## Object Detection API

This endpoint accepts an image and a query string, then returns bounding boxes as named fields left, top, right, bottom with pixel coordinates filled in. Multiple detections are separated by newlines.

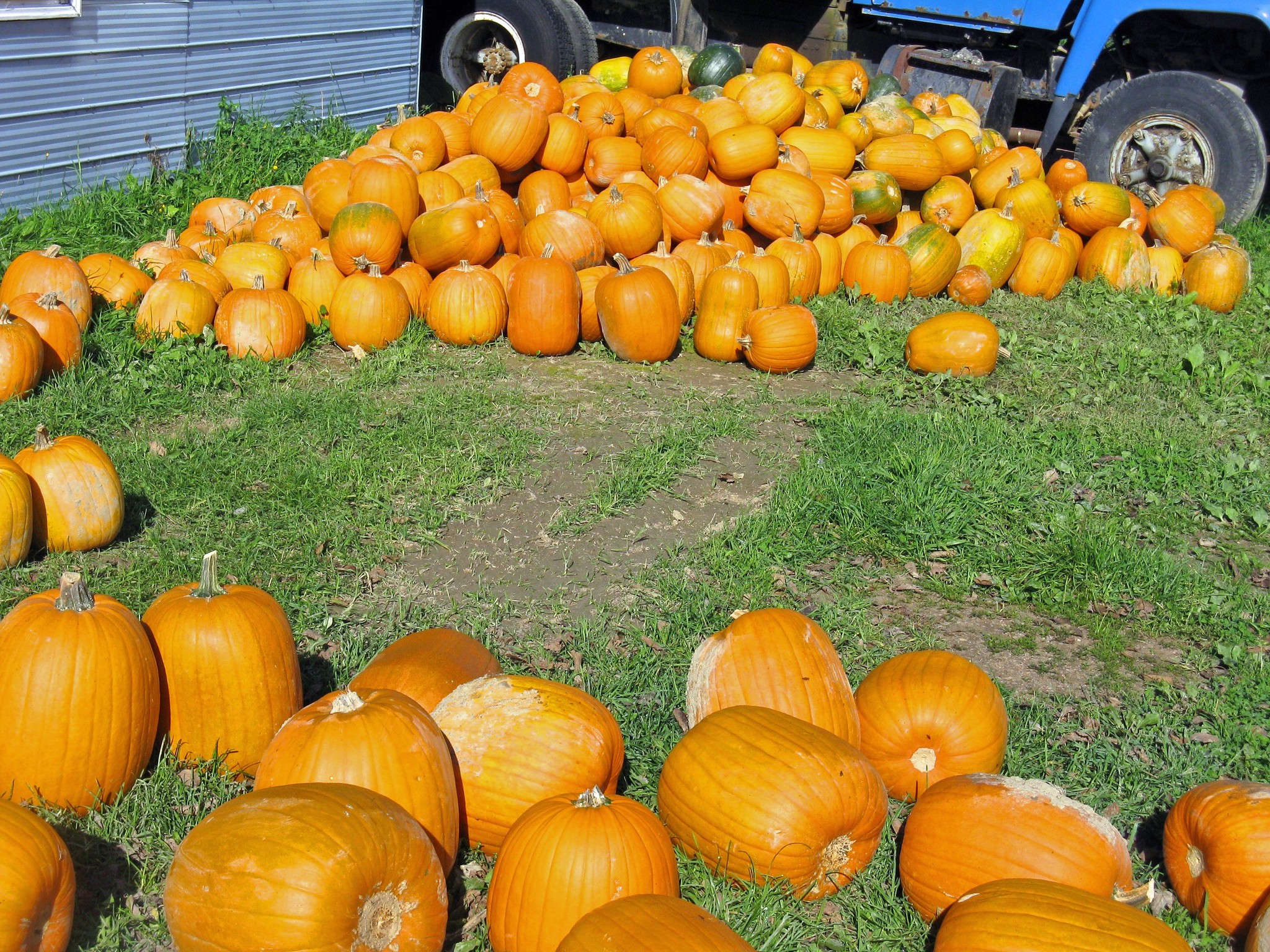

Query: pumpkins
left=428, top=675, right=627, bottom=857
left=656, top=708, right=889, bottom=901
left=140, top=549, right=303, bottom=790
left=12, top=424, right=127, bottom=558
left=161, top=781, right=448, bottom=951
left=933, top=879, right=1193, bottom=952
left=555, top=893, right=753, bottom=951
left=2, top=42, right=1256, bottom=412
left=2, top=803, right=77, bottom=952
left=347, top=627, right=503, bottom=716
left=685, top=608, right=863, bottom=751
left=0, top=453, right=34, bottom=572
left=853, top=648, right=1012, bottom=804
left=898, top=771, right=1157, bottom=927
left=254, top=688, right=463, bottom=877
left=2, top=574, right=164, bottom=823
left=486, top=784, right=681, bottom=951
left=1162, top=774, right=1269, bottom=951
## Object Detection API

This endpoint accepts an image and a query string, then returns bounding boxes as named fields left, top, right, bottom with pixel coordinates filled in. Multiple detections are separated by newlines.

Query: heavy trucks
left=422, top=1, right=1269, bottom=232
